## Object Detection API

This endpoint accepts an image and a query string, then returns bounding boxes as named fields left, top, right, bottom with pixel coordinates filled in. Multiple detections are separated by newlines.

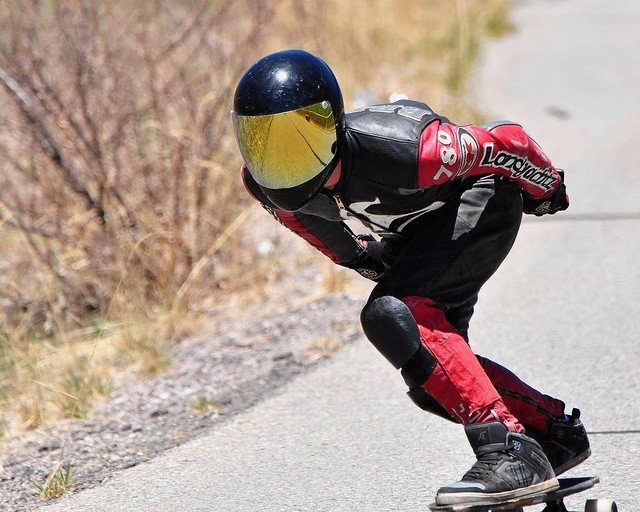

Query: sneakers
left=435, top=421, right=561, bottom=505
left=524, top=408, right=591, bottom=476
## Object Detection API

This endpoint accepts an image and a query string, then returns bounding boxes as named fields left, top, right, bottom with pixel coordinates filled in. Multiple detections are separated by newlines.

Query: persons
left=231, top=48, right=592, bottom=506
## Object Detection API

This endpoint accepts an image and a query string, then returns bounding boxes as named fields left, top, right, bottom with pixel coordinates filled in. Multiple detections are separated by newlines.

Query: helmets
left=231, top=48, right=347, bottom=213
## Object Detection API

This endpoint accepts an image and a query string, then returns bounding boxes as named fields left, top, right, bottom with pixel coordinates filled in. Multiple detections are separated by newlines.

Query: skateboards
left=428, top=476, right=618, bottom=512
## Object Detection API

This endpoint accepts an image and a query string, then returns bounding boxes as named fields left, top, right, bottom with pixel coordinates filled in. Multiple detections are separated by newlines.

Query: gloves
left=336, top=233, right=391, bottom=282
left=521, top=168, right=570, bottom=217
